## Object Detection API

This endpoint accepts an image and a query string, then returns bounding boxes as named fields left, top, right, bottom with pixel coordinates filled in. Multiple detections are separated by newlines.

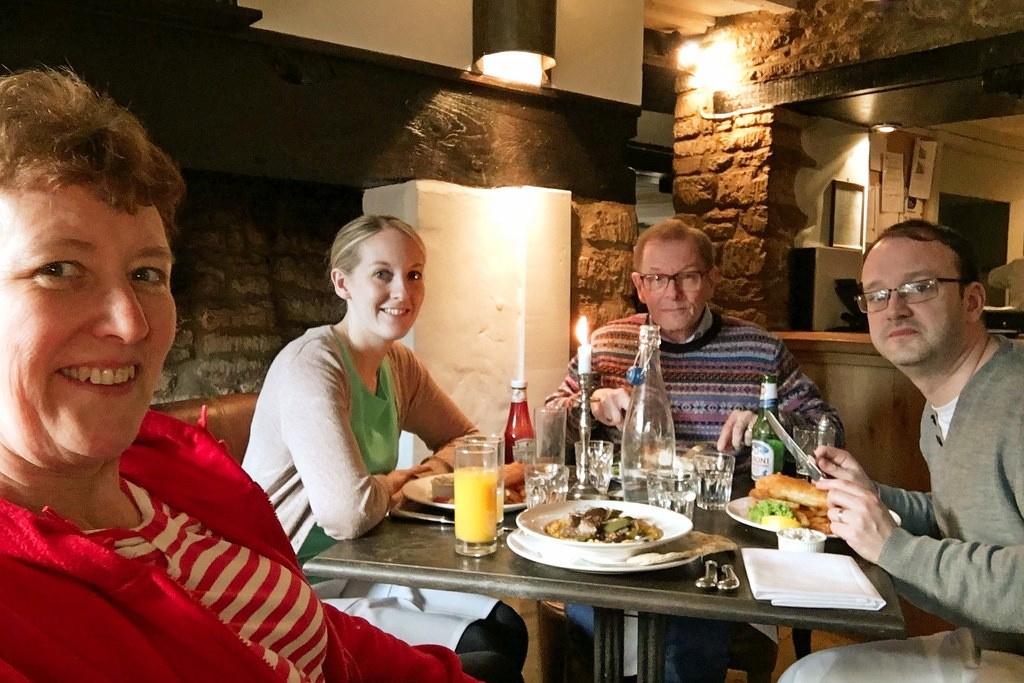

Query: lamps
left=472, top=0, right=557, bottom=87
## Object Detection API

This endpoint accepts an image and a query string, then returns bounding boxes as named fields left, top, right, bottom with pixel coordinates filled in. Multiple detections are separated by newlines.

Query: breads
left=749, top=474, right=828, bottom=507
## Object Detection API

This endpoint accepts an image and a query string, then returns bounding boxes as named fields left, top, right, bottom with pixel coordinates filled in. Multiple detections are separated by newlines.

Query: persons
left=0, top=70, right=515, bottom=683
left=778, top=219, right=1024, bottom=681
left=542, top=218, right=845, bottom=683
left=240, top=214, right=527, bottom=670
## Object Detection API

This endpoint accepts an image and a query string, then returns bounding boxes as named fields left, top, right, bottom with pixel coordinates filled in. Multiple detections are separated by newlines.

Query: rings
left=838, top=508, right=844, bottom=523
left=604, top=417, right=612, bottom=422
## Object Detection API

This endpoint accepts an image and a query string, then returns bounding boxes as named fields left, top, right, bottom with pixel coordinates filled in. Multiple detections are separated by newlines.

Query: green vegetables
left=747, top=501, right=793, bottom=523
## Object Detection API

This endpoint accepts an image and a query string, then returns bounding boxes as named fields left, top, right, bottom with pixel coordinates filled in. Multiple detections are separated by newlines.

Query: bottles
left=621, top=325, right=676, bottom=505
left=751, top=374, right=783, bottom=490
left=504, top=381, right=537, bottom=465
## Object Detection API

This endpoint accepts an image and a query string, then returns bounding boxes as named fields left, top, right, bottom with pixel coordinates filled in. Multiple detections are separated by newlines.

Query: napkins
left=742, top=547, right=886, bottom=610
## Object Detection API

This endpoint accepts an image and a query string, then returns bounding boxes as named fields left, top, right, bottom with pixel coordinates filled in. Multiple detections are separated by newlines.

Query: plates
left=726, top=496, right=901, bottom=537
left=402, top=472, right=527, bottom=513
left=609, top=456, right=697, bottom=483
left=506, top=527, right=700, bottom=574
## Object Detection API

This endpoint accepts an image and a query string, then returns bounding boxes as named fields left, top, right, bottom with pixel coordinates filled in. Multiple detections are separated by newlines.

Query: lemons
left=762, top=515, right=801, bottom=528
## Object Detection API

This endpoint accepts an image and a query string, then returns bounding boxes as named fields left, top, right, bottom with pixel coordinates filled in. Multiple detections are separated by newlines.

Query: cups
left=647, top=470, right=697, bottom=522
left=693, top=452, right=735, bottom=511
left=464, top=435, right=505, bottom=538
left=454, top=443, right=497, bottom=557
left=534, top=407, right=566, bottom=466
left=574, top=440, right=614, bottom=494
left=524, top=463, right=569, bottom=509
left=792, top=425, right=835, bottom=476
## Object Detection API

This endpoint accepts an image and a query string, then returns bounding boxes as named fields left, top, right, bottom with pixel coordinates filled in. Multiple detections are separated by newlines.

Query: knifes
left=388, top=508, right=516, bottom=535
left=764, top=410, right=827, bottom=483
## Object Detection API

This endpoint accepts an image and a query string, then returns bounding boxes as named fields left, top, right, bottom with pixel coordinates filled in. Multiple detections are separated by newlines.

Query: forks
left=795, top=430, right=820, bottom=464
left=683, top=442, right=717, bottom=458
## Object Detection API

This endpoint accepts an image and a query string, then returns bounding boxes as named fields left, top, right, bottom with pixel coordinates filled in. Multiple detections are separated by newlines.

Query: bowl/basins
left=776, top=527, right=826, bottom=553
left=430, top=476, right=454, bottom=502
left=516, top=500, right=693, bottom=562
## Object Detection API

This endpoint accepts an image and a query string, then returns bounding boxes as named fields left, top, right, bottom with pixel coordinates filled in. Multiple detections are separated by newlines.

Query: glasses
left=640, top=265, right=711, bottom=295
left=854, top=277, right=970, bottom=313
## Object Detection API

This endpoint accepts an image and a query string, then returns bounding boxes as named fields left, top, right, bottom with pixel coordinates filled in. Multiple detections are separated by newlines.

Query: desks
left=302, top=463, right=906, bottom=683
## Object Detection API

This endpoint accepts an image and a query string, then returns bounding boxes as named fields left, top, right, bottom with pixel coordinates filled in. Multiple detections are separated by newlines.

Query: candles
left=518, top=313, right=525, bottom=380
left=575, top=316, right=592, bottom=374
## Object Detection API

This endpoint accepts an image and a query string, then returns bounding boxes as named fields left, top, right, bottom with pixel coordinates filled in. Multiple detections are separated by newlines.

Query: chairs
left=148, top=393, right=258, bottom=467
